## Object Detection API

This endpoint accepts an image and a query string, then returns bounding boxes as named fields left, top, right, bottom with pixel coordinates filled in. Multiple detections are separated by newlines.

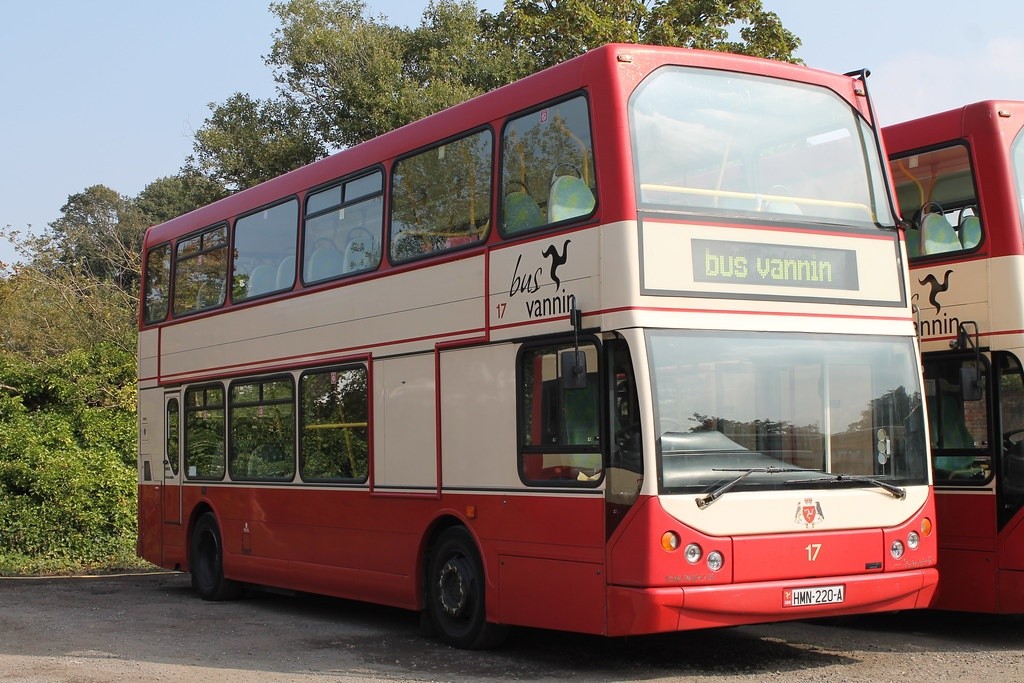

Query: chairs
left=276, top=245, right=299, bottom=290
left=902, top=218, right=919, bottom=259
left=219, top=277, right=227, bottom=307
left=546, top=163, right=597, bottom=227
left=307, top=247, right=334, bottom=285
left=194, top=278, right=218, bottom=310
left=957, top=202, right=981, bottom=252
left=342, top=227, right=378, bottom=274
left=761, top=185, right=800, bottom=214
left=244, top=260, right=278, bottom=298
left=919, top=198, right=963, bottom=258
left=504, top=179, right=544, bottom=241
left=928, top=396, right=985, bottom=481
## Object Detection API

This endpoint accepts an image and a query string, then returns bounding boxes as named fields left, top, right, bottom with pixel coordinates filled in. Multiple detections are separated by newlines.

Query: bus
left=526, top=94, right=1024, bottom=627
left=132, top=36, right=945, bottom=646
